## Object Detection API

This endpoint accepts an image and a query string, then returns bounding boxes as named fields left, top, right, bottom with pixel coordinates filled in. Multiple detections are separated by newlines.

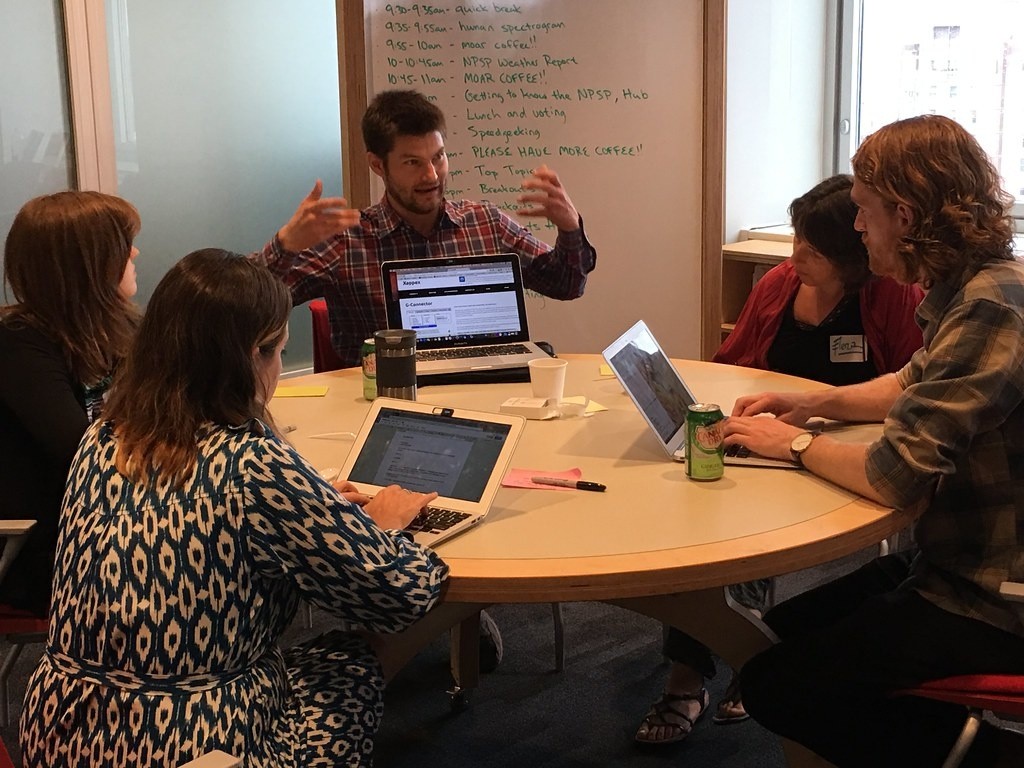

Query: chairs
left=300, top=299, right=565, bottom=673
left=0, top=517, right=50, bottom=768
left=893, top=581, right=1024, bottom=768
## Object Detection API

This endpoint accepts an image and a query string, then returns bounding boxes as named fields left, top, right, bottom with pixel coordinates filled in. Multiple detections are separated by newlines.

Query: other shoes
left=477, top=608, right=503, bottom=674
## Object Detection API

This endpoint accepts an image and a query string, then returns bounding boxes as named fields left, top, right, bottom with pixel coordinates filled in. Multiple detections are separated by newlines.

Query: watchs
left=790, top=429, right=822, bottom=470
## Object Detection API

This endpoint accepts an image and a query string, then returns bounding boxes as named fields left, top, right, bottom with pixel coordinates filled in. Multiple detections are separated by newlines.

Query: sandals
left=635, top=684, right=710, bottom=743
left=713, top=678, right=752, bottom=724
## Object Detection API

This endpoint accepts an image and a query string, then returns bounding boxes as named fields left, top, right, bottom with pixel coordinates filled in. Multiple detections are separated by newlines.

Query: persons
left=247, top=91, right=596, bottom=370
left=20, top=247, right=449, bottom=768
left=0, top=192, right=148, bottom=768
left=636, top=174, right=925, bottom=744
left=722, top=114, right=1024, bottom=768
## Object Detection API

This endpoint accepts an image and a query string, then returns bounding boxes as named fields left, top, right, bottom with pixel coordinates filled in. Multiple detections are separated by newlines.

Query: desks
left=265, top=354, right=934, bottom=768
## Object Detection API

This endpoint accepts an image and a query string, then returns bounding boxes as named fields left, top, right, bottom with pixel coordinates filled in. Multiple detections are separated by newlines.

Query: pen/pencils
left=531, top=475, right=608, bottom=491
left=282, top=424, right=297, bottom=434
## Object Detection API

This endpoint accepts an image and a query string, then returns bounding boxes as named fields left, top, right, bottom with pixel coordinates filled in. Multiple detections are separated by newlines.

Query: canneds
left=685, top=403, right=725, bottom=481
left=362, top=338, right=378, bottom=401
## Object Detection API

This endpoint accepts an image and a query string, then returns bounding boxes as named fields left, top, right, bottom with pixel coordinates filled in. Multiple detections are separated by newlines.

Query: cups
left=374, top=329, right=417, bottom=401
left=527, top=358, right=568, bottom=398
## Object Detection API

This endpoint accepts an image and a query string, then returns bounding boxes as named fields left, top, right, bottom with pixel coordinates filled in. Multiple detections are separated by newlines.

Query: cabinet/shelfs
left=721, top=239, right=793, bottom=333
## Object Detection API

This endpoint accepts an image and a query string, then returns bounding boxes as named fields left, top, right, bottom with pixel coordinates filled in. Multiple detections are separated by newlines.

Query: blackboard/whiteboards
left=336, top=0, right=728, bottom=362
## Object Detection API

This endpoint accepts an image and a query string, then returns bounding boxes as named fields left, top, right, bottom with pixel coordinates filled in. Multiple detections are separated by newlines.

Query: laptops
left=335, top=397, right=526, bottom=550
left=380, top=252, right=550, bottom=376
left=602, top=320, right=825, bottom=469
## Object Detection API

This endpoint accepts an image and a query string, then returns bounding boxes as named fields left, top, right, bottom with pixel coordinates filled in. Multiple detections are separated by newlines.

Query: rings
left=402, top=488, right=412, bottom=495
